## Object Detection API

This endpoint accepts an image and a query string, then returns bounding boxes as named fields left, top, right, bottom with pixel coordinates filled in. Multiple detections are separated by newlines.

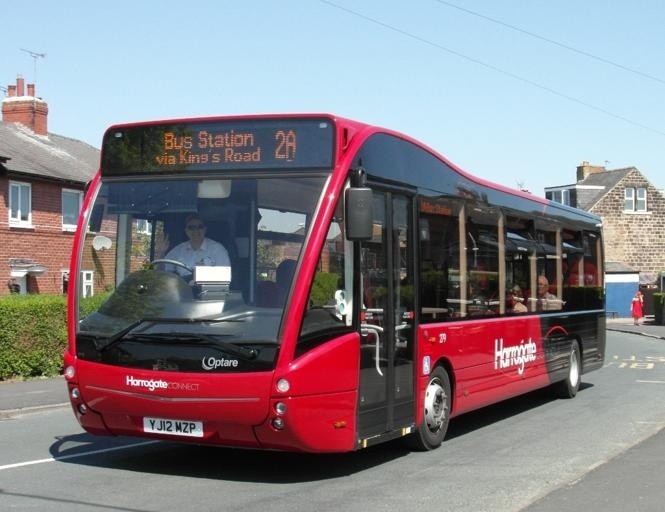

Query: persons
left=506, top=283, right=530, bottom=314
left=159, top=210, right=231, bottom=288
left=526, top=276, right=561, bottom=312
left=7, top=278, right=21, bottom=294
left=468, top=289, right=497, bottom=318
left=630, top=291, right=644, bottom=326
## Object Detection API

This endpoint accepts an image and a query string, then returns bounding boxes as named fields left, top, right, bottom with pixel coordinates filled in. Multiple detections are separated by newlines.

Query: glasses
left=187, top=224, right=206, bottom=230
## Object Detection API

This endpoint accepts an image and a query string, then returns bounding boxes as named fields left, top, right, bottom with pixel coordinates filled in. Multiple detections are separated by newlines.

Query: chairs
left=521, top=256, right=598, bottom=311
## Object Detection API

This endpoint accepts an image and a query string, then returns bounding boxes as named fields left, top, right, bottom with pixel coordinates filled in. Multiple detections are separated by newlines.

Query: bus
left=61, top=110, right=610, bottom=460
